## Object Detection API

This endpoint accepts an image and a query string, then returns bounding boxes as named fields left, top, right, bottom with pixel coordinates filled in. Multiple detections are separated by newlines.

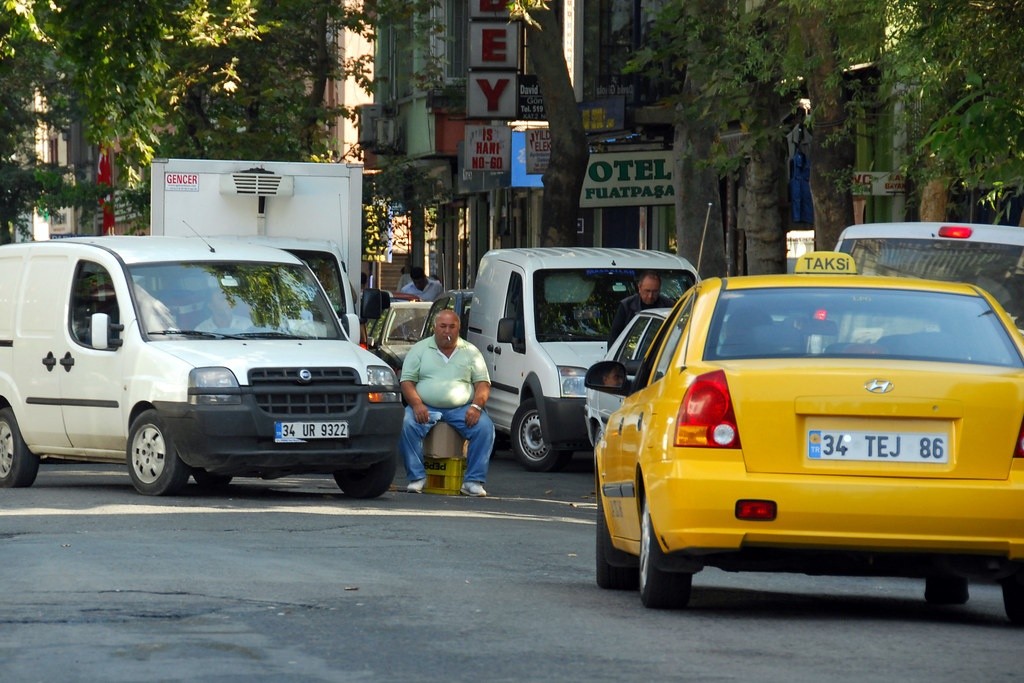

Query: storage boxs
left=422, top=421, right=469, bottom=495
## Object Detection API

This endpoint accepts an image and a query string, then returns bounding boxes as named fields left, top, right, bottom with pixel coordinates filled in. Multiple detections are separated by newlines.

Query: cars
left=581, top=250, right=1024, bottom=627
left=365, top=302, right=432, bottom=405
left=418, top=288, right=474, bottom=343
left=582, top=307, right=686, bottom=452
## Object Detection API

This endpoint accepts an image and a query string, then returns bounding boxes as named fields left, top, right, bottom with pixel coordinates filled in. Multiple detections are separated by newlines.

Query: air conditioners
left=356, top=102, right=382, bottom=145
left=373, top=117, right=395, bottom=147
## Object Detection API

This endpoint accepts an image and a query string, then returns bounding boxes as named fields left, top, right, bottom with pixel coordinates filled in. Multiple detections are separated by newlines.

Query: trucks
left=150, top=153, right=365, bottom=351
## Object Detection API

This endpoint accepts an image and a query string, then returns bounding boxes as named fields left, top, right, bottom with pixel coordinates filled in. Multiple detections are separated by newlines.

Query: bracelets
left=471, top=404, right=482, bottom=412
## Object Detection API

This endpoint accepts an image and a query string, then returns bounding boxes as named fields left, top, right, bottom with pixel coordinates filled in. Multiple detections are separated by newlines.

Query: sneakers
left=460, top=481, right=486, bottom=496
left=407, top=478, right=425, bottom=493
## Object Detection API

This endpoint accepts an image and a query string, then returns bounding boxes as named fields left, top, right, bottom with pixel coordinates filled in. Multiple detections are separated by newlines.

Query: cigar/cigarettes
left=448, top=335, right=451, bottom=341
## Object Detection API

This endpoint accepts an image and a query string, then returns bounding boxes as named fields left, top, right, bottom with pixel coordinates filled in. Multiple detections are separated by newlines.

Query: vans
left=0, top=236, right=407, bottom=501
left=831, top=222, right=1023, bottom=351
left=466, top=247, right=703, bottom=475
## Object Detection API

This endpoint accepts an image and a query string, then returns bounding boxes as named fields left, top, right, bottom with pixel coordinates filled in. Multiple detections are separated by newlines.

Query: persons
left=194, top=288, right=254, bottom=334
left=398, top=309, right=495, bottom=495
left=397, top=267, right=444, bottom=302
left=602, top=365, right=624, bottom=386
left=608, top=273, right=673, bottom=352
left=360, top=272, right=366, bottom=344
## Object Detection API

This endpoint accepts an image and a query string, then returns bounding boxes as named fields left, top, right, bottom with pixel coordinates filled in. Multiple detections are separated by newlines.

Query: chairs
left=721, top=307, right=782, bottom=357
left=774, top=316, right=838, bottom=354
left=825, top=342, right=890, bottom=357
left=86, top=282, right=117, bottom=319
